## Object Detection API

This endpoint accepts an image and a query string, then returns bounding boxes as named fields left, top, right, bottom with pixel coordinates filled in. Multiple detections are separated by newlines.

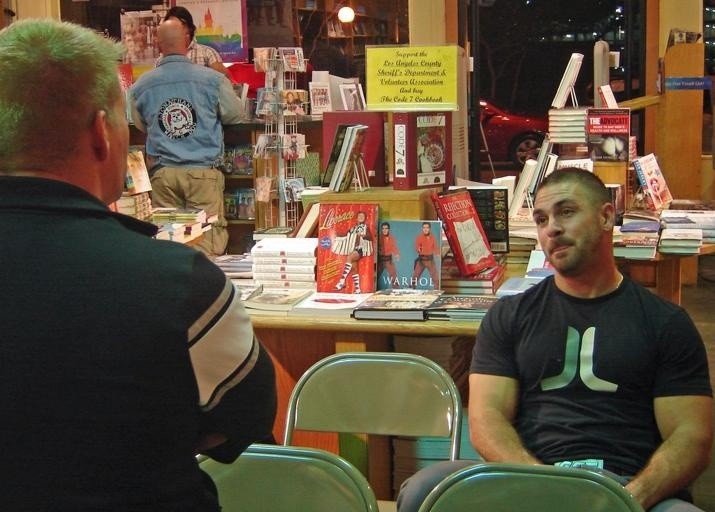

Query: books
left=338, top=83, right=368, bottom=110
left=303, top=1, right=387, bottom=58
left=253, top=45, right=309, bottom=206
left=549, top=51, right=585, bottom=109
left=336, top=331, right=485, bottom=511
left=307, top=81, right=333, bottom=115
left=196, top=101, right=715, bottom=322
left=104, top=150, right=219, bottom=243
left=597, top=84, right=621, bottom=109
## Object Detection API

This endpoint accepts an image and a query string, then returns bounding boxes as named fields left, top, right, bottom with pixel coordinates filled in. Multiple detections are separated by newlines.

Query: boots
left=334, top=264, right=351, bottom=291
left=353, top=274, right=360, bottom=293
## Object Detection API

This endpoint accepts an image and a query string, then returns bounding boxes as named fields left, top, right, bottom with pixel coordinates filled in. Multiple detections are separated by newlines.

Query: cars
left=478, top=93, right=550, bottom=172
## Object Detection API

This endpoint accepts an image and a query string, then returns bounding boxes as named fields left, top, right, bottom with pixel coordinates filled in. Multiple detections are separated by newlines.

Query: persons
left=126, top=18, right=247, bottom=257
left=395, top=168, right=715, bottom=510
left=154, top=5, right=228, bottom=77
left=0, top=14, right=279, bottom=511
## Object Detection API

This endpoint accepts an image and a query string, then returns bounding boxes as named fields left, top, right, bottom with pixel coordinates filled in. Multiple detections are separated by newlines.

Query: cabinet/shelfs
left=220, top=121, right=266, bottom=229
left=294, top=1, right=401, bottom=77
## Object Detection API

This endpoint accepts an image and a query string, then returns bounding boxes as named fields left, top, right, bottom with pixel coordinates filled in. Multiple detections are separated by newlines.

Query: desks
left=248, top=259, right=532, bottom=498
